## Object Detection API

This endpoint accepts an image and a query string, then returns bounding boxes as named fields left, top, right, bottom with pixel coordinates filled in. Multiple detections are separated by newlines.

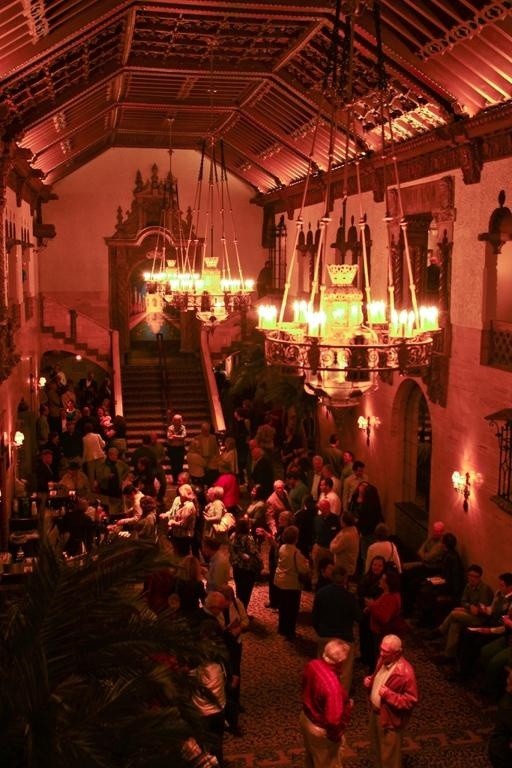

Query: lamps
left=32, top=221, right=58, bottom=255
left=450, top=467, right=476, bottom=514
left=357, top=412, right=379, bottom=448
left=33, top=375, right=48, bottom=391
left=167, top=34, right=262, bottom=336
left=10, top=430, right=29, bottom=453
left=140, top=112, right=201, bottom=302
left=254, top=2, right=446, bottom=422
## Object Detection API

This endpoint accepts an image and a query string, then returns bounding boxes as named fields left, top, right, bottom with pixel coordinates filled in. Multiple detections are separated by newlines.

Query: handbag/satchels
left=300, top=573, right=313, bottom=583
left=213, top=511, right=238, bottom=534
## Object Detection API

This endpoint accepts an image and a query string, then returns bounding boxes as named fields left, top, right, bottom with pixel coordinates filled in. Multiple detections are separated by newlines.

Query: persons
left=487, top=664, right=511, bottom=768
left=297, top=639, right=353, bottom=768
left=363, top=635, right=418, bottom=768
left=0, top=361, right=511, bottom=737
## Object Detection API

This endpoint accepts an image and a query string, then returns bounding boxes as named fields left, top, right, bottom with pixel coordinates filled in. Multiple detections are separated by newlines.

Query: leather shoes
left=432, top=653, right=454, bottom=665
left=426, top=626, right=443, bottom=639
left=231, top=726, right=244, bottom=738
